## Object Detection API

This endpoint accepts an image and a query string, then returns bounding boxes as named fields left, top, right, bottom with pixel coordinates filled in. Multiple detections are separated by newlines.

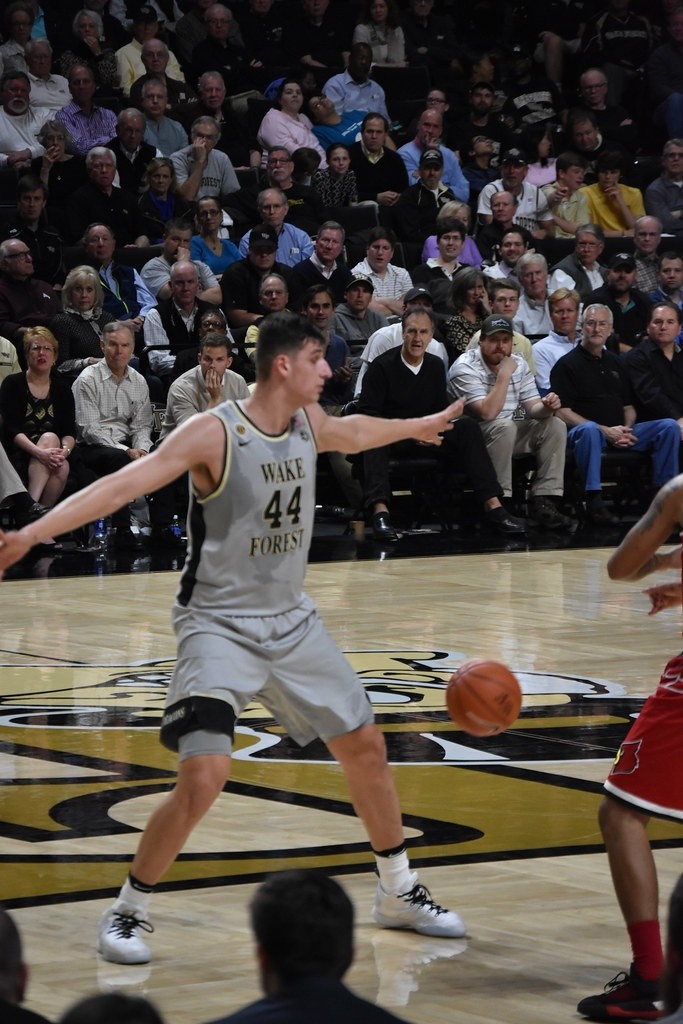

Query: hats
left=132, top=5, right=157, bottom=24
left=345, top=275, right=374, bottom=293
left=420, top=149, right=443, bottom=166
left=481, top=314, right=513, bottom=338
left=501, top=148, right=526, bottom=164
left=404, top=288, right=434, bottom=305
left=608, top=252, right=636, bottom=270
left=250, top=224, right=278, bottom=247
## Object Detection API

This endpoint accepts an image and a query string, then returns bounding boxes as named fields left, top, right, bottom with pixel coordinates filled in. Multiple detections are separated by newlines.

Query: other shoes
left=149, top=523, right=172, bottom=553
left=11, top=502, right=52, bottom=531
left=527, top=503, right=578, bottom=531
left=113, top=529, right=142, bottom=551
left=585, top=503, right=619, bottom=528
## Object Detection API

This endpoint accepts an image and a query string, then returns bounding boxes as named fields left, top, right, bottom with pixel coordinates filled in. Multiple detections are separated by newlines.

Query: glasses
left=426, top=98, right=446, bottom=105
left=313, top=95, right=327, bottom=109
left=611, top=263, right=634, bottom=273
left=201, top=321, right=225, bottom=329
left=268, top=157, right=292, bottom=165
left=199, top=209, right=220, bottom=218
left=3, top=250, right=31, bottom=260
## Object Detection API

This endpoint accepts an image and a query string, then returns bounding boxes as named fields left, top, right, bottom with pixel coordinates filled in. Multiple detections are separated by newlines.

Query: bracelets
left=62, top=446, right=71, bottom=455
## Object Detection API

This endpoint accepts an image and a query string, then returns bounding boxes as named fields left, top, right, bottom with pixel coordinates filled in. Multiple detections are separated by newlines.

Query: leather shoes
left=375, top=513, right=396, bottom=538
left=482, top=515, right=525, bottom=534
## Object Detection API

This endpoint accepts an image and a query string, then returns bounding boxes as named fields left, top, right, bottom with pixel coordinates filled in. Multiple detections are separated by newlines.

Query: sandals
left=37, top=540, right=64, bottom=550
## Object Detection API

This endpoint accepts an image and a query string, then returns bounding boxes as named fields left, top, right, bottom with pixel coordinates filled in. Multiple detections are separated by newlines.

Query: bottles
left=95, top=516, right=108, bottom=561
left=169, top=514, right=182, bottom=541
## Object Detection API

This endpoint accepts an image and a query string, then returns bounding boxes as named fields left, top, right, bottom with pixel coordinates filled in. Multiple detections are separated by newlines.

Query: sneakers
left=96, top=902, right=154, bottom=964
left=576, top=963, right=679, bottom=1024
left=98, top=958, right=151, bottom=991
left=371, top=929, right=467, bottom=985
left=371, top=868, right=467, bottom=938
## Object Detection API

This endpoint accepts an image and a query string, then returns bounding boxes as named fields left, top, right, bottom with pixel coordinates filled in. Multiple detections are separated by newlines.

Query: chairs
left=338, top=451, right=650, bottom=530
left=383, top=66, right=432, bottom=126
left=0, top=143, right=411, bottom=274
left=530, top=234, right=683, bottom=270
left=624, top=155, right=661, bottom=196
left=621, top=65, right=670, bottom=155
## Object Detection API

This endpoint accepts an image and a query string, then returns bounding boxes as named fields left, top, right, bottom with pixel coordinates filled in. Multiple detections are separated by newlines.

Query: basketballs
left=445, top=659, right=522, bottom=736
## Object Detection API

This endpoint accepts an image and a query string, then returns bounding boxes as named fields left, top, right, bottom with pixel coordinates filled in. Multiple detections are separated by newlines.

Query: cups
left=350, top=521, right=365, bottom=536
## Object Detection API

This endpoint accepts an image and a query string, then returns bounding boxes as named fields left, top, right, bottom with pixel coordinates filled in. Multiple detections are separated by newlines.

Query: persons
left=0, top=0, right=683, bottom=558
left=0, top=311, right=466, bottom=965
left=576, top=474, right=683, bottom=1024
left=0, top=869, right=411, bottom=1024
